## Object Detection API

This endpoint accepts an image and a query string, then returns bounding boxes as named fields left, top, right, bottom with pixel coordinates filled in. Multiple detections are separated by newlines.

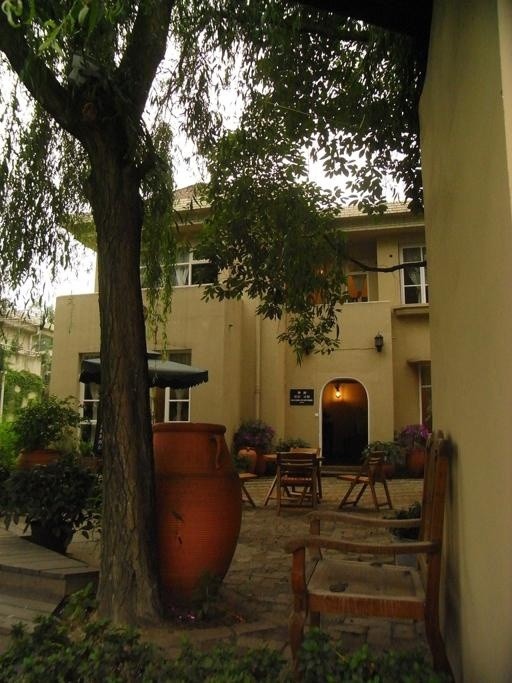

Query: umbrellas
left=78, top=351, right=208, bottom=390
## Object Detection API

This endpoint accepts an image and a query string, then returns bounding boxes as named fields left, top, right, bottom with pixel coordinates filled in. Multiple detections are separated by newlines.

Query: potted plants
left=381, top=499, right=422, bottom=566
left=363, top=439, right=401, bottom=478
left=1, top=452, right=105, bottom=554
left=11, top=392, right=91, bottom=470
left=77, top=439, right=98, bottom=470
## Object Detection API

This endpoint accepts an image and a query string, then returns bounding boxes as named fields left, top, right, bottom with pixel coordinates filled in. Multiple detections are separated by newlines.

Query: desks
left=261, top=453, right=328, bottom=506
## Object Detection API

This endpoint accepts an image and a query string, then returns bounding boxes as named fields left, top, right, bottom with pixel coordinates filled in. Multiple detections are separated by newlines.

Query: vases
left=405, top=448, right=428, bottom=478
left=236, top=447, right=259, bottom=473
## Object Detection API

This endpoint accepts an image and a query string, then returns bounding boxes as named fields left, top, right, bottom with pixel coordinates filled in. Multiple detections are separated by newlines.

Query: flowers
left=394, top=423, right=430, bottom=447
left=233, top=418, right=276, bottom=447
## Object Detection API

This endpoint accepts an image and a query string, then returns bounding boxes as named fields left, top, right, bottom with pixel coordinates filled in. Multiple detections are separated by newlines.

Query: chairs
left=238, top=471, right=259, bottom=507
left=289, top=445, right=320, bottom=500
left=286, top=428, right=453, bottom=681
left=275, top=451, right=319, bottom=516
left=335, top=449, right=394, bottom=511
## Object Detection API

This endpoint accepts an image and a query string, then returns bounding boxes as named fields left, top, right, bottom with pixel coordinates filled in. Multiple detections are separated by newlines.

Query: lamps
left=333, top=381, right=343, bottom=397
left=375, top=329, right=384, bottom=352
